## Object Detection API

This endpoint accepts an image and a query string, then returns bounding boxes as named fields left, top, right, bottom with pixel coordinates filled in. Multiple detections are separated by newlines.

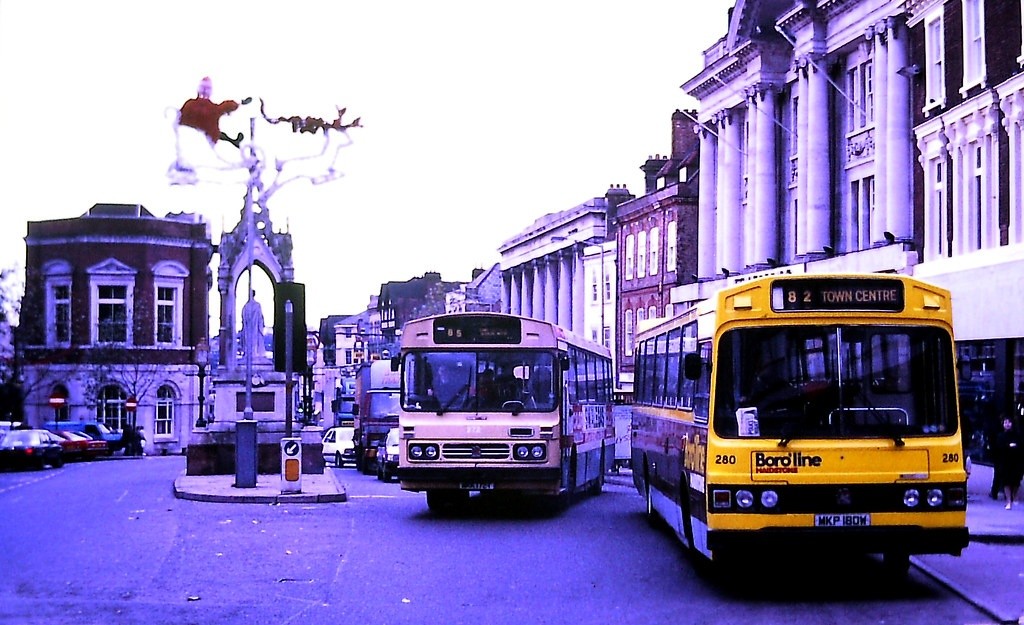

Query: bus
left=630, top=274, right=971, bottom=579
left=389, top=311, right=616, bottom=514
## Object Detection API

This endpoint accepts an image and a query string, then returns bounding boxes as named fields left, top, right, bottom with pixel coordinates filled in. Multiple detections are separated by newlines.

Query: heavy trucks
left=332, top=381, right=358, bottom=426
left=353, top=361, right=403, bottom=475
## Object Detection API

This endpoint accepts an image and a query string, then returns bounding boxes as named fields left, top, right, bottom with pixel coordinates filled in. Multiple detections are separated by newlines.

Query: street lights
left=186, top=337, right=217, bottom=476
left=549, top=236, right=604, bottom=345
left=298, top=352, right=323, bottom=475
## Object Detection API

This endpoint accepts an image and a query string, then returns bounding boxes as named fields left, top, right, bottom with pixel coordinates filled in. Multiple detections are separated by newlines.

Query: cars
left=51, top=430, right=109, bottom=461
left=1, top=429, right=64, bottom=472
left=322, top=427, right=358, bottom=467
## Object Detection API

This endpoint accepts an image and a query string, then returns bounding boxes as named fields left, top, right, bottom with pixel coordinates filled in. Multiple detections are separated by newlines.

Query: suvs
left=376, top=428, right=403, bottom=483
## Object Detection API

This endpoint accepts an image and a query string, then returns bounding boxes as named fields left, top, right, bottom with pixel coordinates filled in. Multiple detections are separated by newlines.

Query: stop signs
left=125, top=397, right=137, bottom=412
left=48, top=390, right=65, bottom=408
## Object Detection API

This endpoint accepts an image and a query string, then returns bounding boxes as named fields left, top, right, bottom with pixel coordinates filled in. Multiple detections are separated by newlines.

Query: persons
left=240, top=290, right=268, bottom=359
left=428, top=358, right=552, bottom=407
left=986, top=415, right=1024, bottom=510
left=121, top=424, right=148, bottom=456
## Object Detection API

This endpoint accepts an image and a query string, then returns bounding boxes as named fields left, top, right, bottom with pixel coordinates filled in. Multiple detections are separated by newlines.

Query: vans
left=46, top=421, right=124, bottom=457
